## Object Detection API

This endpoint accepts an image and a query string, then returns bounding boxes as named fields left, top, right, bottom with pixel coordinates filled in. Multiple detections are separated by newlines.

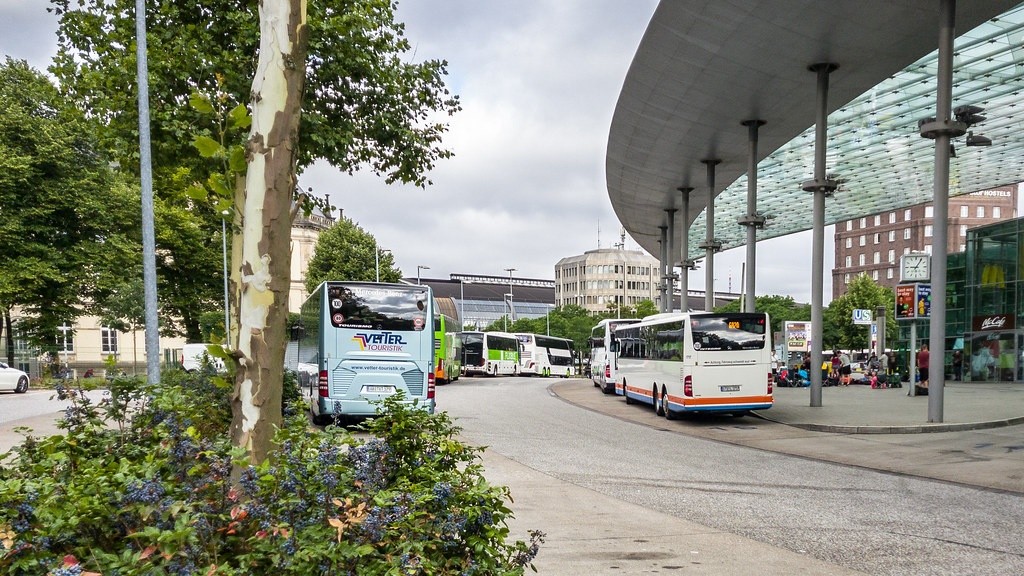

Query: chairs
left=788, top=368, right=803, bottom=387
left=822, top=369, right=829, bottom=387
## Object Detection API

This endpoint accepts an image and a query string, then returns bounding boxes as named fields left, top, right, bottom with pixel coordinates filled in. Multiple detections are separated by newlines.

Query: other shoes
left=846, top=383, right=849, bottom=387
left=841, top=383, right=844, bottom=386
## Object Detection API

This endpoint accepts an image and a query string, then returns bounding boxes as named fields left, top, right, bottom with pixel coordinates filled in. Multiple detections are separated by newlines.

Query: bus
left=514, top=333, right=576, bottom=378
left=463, top=332, right=525, bottom=377
left=435, top=315, right=463, bottom=384
left=590, top=308, right=774, bottom=421
left=298, top=280, right=436, bottom=427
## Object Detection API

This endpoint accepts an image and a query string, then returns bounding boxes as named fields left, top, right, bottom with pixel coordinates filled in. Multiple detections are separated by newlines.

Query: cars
left=0, top=362, right=30, bottom=394
left=584, top=357, right=591, bottom=378
left=849, top=362, right=868, bottom=383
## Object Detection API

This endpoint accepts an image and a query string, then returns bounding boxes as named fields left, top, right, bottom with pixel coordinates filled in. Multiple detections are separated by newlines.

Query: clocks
left=900, top=253, right=930, bottom=282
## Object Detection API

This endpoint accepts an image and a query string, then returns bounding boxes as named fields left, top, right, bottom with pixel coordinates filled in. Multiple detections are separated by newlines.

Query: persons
left=805, top=354, right=811, bottom=381
left=918, top=344, right=929, bottom=387
left=952, top=351, right=962, bottom=381
left=919, top=297, right=925, bottom=315
left=897, top=295, right=908, bottom=317
left=830, top=349, right=852, bottom=386
left=771, top=350, right=787, bottom=385
left=84, top=368, right=94, bottom=378
left=864, top=352, right=897, bottom=388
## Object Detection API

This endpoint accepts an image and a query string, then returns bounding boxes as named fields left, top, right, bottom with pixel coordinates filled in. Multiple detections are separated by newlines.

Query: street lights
left=418, top=265, right=431, bottom=285
left=376, top=246, right=392, bottom=282
left=458, top=277, right=471, bottom=330
left=504, top=292, right=514, bottom=333
left=221, top=210, right=230, bottom=349
left=504, top=268, right=518, bottom=325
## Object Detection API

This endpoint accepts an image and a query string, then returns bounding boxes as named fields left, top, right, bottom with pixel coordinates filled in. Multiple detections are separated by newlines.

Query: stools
left=876, top=380, right=887, bottom=389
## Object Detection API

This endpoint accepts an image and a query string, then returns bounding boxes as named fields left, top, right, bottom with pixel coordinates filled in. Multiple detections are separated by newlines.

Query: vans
left=182, top=342, right=231, bottom=375
